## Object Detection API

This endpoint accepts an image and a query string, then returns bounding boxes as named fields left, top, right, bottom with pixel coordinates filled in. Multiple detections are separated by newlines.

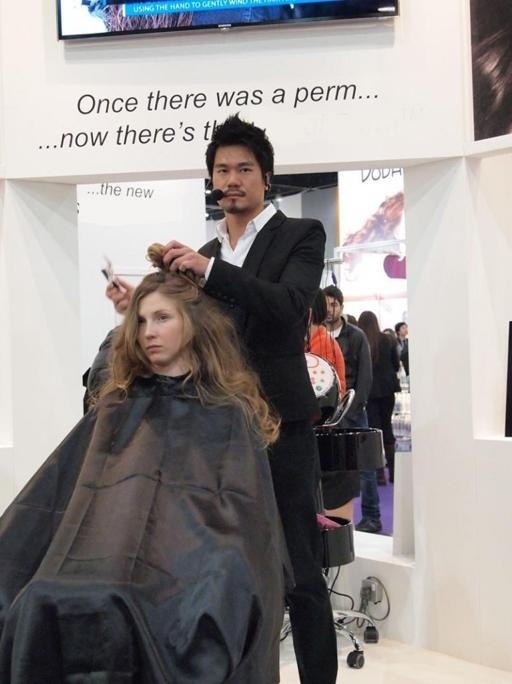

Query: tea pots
left=77, top=167, right=411, bottom=536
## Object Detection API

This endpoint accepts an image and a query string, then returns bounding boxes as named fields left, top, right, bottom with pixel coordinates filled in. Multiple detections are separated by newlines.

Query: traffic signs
left=360, top=580, right=384, bottom=605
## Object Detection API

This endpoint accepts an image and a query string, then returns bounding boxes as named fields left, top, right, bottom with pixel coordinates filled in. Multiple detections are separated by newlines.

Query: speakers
left=384, top=444, right=397, bottom=483
left=377, top=468, right=386, bottom=486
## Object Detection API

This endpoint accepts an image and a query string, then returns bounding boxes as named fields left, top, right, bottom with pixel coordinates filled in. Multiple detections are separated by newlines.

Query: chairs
left=355, top=521, right=381, bottom=532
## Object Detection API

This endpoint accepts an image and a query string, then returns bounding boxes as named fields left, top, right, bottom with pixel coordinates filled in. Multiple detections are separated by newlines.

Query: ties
left=55, top=0, right=400, bottom=41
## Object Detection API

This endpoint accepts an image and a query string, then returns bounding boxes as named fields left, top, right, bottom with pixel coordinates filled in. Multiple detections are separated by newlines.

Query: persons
left=305, top=286, right=409, bottom=532
left=0, top=243, right=296, bottom=684
left=106, top=113, right=338, bottom=684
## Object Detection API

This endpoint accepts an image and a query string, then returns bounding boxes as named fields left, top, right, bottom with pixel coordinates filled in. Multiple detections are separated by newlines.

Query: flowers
left=205, top=188, right=227, bottom=201
